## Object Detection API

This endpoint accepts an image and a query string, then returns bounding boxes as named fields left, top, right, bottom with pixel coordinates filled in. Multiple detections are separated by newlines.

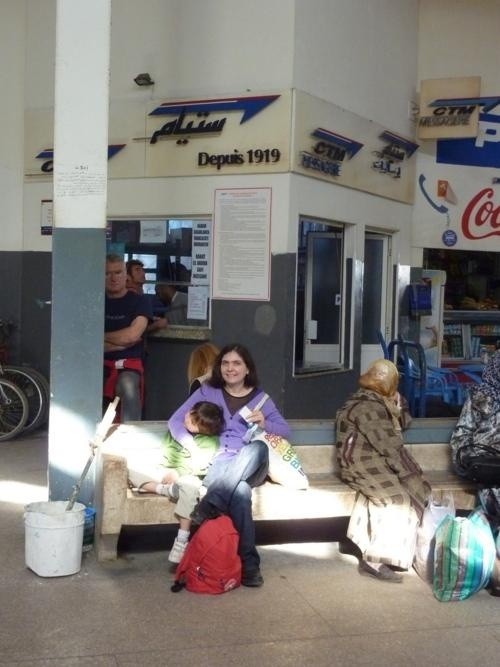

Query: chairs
left=378, top=330, right=463, bottom=409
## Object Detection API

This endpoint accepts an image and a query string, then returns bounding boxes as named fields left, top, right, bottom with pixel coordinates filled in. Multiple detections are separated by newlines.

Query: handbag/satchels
left=250, top=429, right=310, bottom=490
left=467, top=455, right=500, bottom=488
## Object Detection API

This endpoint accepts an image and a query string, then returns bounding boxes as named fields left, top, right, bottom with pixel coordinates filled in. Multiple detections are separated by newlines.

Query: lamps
left=134, top=72, right=152, bottom=86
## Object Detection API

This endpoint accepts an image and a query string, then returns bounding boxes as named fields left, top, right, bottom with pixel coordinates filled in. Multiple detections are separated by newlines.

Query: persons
left=126, top=402, right=224, bottom=562
left=124, top=259, right=147, bottom=296
left=102, top=253, right=154, bottom=423
left=187, top=343, right=222, bottom=398
left=153, top=277, right=189, bottom=324
left=166, top=342, right=291, bottom=587
left=332, top=360, right=432, bottom=582
left=449, top=348, right=500, bottom=592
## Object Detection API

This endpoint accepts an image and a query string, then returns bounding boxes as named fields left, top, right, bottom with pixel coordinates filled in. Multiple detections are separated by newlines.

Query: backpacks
left=175, top=511, right=242, bottom=596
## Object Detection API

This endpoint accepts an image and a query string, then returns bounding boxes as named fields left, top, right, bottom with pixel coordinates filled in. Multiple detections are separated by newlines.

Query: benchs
left=94, top=414, right=482, bottom=562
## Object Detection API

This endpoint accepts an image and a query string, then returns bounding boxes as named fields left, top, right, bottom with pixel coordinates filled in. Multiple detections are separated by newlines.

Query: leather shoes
left=359, top=559, right=404, bottom=583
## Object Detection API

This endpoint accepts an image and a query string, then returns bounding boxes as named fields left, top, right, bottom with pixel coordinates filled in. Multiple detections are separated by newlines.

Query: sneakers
left=167, top=536, right=189, bottom=564
left=166, top=483, right=180, bottom=502
left=241, top=569, right=263, bottom=587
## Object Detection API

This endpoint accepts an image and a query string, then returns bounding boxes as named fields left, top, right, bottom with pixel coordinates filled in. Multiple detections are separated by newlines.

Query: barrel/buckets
left=24, top=500, right=86, bottom=577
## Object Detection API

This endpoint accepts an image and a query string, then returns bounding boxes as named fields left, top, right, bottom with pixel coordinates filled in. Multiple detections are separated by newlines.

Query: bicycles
left=0, top=319, right=50, bottom=444
